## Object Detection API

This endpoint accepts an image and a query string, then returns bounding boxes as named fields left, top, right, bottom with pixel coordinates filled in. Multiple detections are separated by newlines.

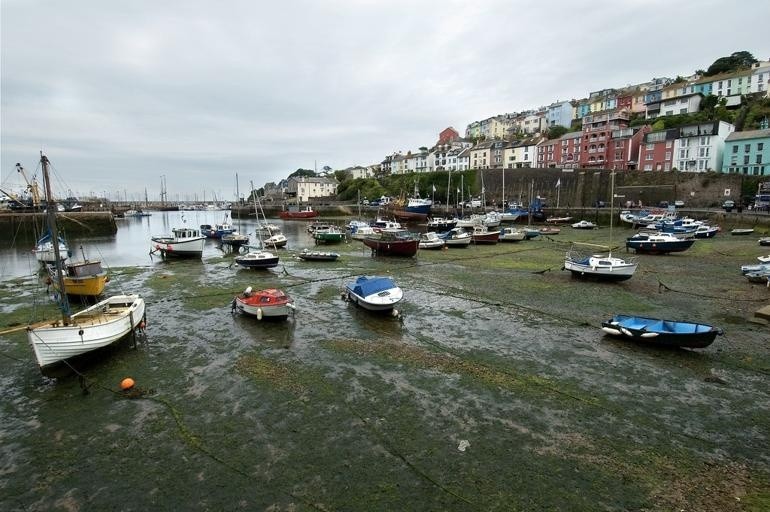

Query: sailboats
left=25, top=156, right=147, bottom=380
left=563, top=164, right=640, bottom=280
left=0, top=150, right=287, bottom=272
left=345, top=165, right=574, bottom=238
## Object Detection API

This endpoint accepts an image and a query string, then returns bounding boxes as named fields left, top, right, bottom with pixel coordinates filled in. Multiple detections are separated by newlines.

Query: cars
left=592, top=200, right=604, bottom=208
left=674, top=200, right=685, bottom=208
left=655, top=201, right=668, bottom=208
left=623, top=200, right=642, bottom=208
left=722, top=200, right=735, bottom=209
left=747, top=203, right=753, bottom=211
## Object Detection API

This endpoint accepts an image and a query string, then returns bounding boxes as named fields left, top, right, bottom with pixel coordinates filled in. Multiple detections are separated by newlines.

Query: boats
left=278, top=196, right=317, bottom=219
left=436, top=225, right=471, bottom=248
left=497, top=226, right=527, bottom=242
left=467, top=217, right=501, bottom=245
left=299, top=248, right=339, bottom=262
left=362, top=224, right=421, bottom=257
left=418, top=231, right=445, bottom=251
left=342, top=274, right=404, bottom=311
left=731, top=228, right=754, bottom=235
left=619, top=207, right=720, bottom=258
left=741, top=236, right=770, bottom=289
left=234, top=286, right=294, bottom=319
left=601, top=311, right=724, bottom=350
left=49, top=243, right=108, bottom=298
left=305, top=219, right=347, bottom=245
left=571, top=219, right=597, bottom=230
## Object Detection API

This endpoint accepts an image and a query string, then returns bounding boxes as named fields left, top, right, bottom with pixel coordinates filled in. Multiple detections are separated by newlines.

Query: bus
left=753, top=194, right=770, bottom=211
